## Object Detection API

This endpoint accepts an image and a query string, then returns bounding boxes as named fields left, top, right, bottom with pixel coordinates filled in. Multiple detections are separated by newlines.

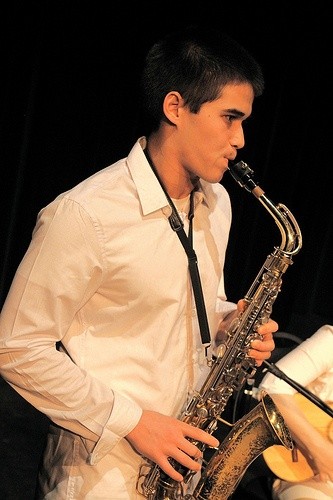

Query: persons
left=255, top=324, right=332, bottom=499
left=0, top=37, right=279, bottom=500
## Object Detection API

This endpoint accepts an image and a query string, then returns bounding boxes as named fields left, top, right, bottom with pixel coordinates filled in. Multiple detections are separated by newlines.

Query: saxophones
left=135, top=159, right=294, bottom=500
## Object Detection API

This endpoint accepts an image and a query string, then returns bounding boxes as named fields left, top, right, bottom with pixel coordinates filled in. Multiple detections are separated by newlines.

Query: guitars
left=246, top=393, right=333, bottom=483
